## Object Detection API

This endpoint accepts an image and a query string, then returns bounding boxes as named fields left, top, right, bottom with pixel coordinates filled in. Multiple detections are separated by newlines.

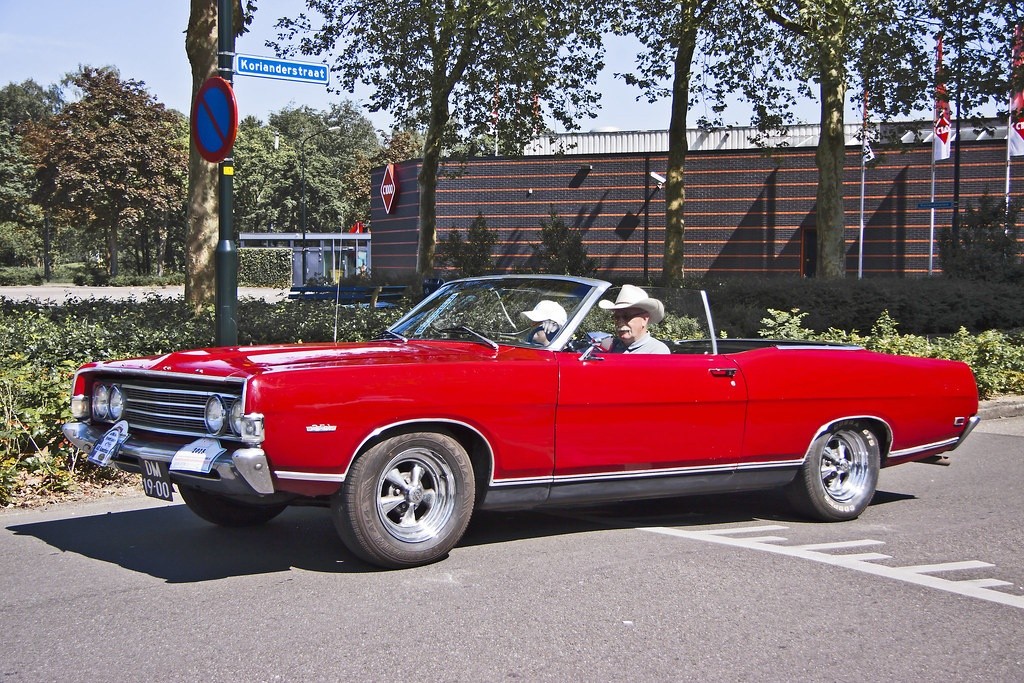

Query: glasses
left=529, top=322, right=542, bottom=327
left=610, top=311, right=648, bottom=323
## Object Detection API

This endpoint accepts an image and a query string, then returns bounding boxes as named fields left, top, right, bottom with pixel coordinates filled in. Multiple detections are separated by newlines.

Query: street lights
left=301, top=126, right=341, bottom=288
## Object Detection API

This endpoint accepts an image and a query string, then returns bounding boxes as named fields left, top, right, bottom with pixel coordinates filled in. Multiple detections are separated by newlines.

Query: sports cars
left=64, top=274, right=982, bottom=567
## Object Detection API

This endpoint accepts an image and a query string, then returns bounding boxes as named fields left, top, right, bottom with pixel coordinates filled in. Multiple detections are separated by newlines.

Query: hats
left=520, top=300, right=567, bottom=327
left=599, top=284, right=665, bottom=324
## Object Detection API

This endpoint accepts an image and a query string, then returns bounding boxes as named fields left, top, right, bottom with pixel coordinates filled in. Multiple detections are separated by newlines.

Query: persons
left=519, top=300, right=567, bottom=348
left=598, top=284, right=674, bottom=354
